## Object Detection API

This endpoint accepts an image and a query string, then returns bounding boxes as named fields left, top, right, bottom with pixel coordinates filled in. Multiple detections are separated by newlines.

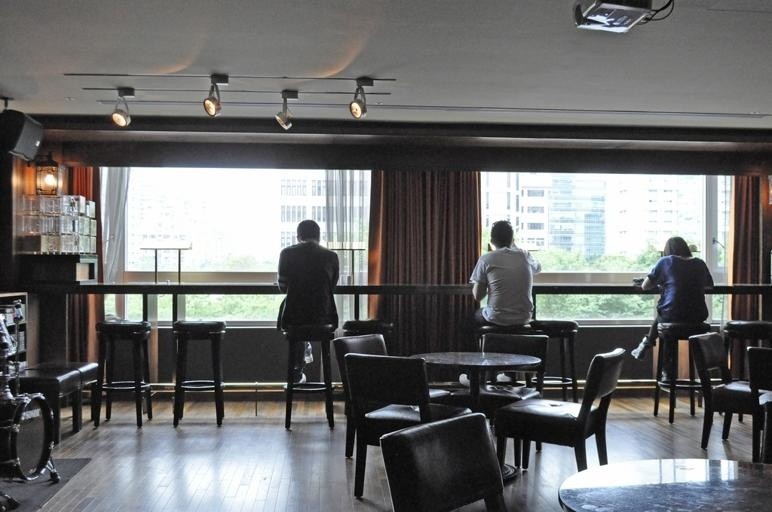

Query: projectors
left=571, top=0, right=654, bottom=36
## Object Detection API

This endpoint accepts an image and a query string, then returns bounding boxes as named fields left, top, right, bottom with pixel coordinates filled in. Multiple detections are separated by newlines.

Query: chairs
left=495, top=348, right=626, bottom=473
left=747, top=346, right=772, bottom=462
left=344, top=352, right=471, bottom=498
left=689, top=332, right=767, bottom=448
left=379, top=412, right=507, bottom=511
left=449, top=332, right=549, bottom=451
left=333, top=333, right=449, bottom=459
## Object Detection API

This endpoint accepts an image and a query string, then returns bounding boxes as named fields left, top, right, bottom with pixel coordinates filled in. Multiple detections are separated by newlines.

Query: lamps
left=113, top=87, right=137, bottom=126
left=349, top=77, right=373, bottom=118
left=275, top=90, right=300, bottom=130
left=25, top=153, right=64, bottom=195
left=203, top=72, right=230, bottom=116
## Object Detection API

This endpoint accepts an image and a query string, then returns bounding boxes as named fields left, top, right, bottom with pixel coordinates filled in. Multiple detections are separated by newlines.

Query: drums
left=0, top=314, right=16, bottom=361
left=0, top=391, right=60, bottom=484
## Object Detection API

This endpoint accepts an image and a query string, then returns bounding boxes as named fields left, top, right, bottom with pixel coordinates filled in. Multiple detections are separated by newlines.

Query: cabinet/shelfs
left=0, top=292, right=27, bottom=381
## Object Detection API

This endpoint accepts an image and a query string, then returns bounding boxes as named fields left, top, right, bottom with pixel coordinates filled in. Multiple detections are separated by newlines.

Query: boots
left=661, top=357, right=675, bottom=382
left=629, top=335, right=652, bottom=360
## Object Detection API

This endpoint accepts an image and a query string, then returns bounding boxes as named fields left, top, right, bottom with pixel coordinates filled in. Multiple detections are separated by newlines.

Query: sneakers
left=305, top=342, right=313, bottom=363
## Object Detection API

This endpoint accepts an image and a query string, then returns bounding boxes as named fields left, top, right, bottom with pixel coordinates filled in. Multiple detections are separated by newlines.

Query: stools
left=172, top=320, right=227, bottom=427
left=25, top=361, right=100, bottom=444
left=723, top=318, right=771, bottom=357
left=653, top=323, right=710, bottom=425
left=530, top=318, right=579, bottom=404
left=90, top=320, right=152, bottom=427
left=342, top=319, right=397, bottom=358
left=283, top=324, right=335, bottom=431
left=474, top=325, right=532, bottom=385
left=9, top=370, right=80, bottom=445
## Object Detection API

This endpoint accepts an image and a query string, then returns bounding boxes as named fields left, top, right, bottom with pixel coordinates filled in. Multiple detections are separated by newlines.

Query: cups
left=341, top=276, right=352, bottom=285
left=632, top=278, right=644, bottom=287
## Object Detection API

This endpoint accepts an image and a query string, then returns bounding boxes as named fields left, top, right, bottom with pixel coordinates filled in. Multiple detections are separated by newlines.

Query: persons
left=277, top=220, right=339, bottom=386
left=631, top=237, right=714, bottom=383
left=456, top=220, right=541, bottom=388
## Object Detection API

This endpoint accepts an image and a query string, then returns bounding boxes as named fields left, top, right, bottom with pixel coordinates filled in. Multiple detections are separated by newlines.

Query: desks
left=559, top=459, right=772, bottom=512
left=415, top=353, right=538, bottom=482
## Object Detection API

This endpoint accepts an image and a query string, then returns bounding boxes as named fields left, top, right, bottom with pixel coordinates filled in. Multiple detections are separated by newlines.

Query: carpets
left=1, top=459, right=87, bottom=512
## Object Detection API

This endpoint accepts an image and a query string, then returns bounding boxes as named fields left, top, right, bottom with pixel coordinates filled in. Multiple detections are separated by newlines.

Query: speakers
left=0, top=109, right=45, bottom=163
